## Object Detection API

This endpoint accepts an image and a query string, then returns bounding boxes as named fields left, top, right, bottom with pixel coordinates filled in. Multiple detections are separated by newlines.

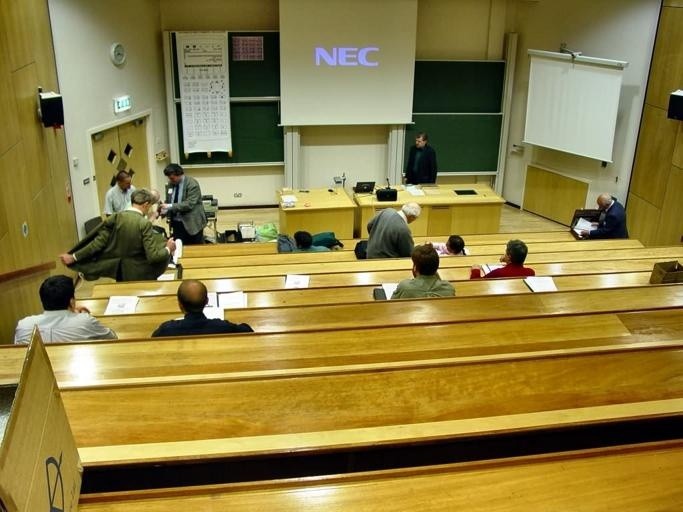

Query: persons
left=125, top=189, right=160, bottom=223
left=103, top=169, right=135, bottom=218
left=403, top=131, right=438, bottom=185
left=580, top=192, right=629, bottom=240
left=58, top=188, right=176, bottom=283
left=149, top=279, right=255, bottom=337
left=470, top=238, right=536, bottom=279
left=430, top=234, right=469, bottom=256
left=13, top=274, right=118, bottom=344
left=390, top=244, right=456, bottom=299
left=366, top=201, right=421, bottom=259
left=160, top=163, right=209, bottom=246
left=291, top=230, right=330, bottom=252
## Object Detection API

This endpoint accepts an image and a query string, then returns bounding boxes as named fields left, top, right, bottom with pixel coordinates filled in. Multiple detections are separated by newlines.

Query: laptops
left=353, top=182, right=375, bottom=192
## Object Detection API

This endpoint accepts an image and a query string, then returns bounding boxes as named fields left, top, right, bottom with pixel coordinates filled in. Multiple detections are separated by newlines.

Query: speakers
left=667, top=90, right=683, bottom=121
left=40, top=92, right=65, bottom=127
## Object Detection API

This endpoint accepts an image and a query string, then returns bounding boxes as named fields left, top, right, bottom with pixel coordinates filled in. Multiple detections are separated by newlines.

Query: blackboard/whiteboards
left=403, top=59, right=506, bottom=176
left=170, top=30, right=283, bottom=168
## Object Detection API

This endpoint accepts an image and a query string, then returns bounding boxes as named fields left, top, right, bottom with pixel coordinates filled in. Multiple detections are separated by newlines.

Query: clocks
left=110, top=42, right=126, bottom=65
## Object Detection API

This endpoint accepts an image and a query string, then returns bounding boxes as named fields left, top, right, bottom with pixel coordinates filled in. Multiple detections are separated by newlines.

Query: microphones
left=386, top=178, right=390, bottom=188
